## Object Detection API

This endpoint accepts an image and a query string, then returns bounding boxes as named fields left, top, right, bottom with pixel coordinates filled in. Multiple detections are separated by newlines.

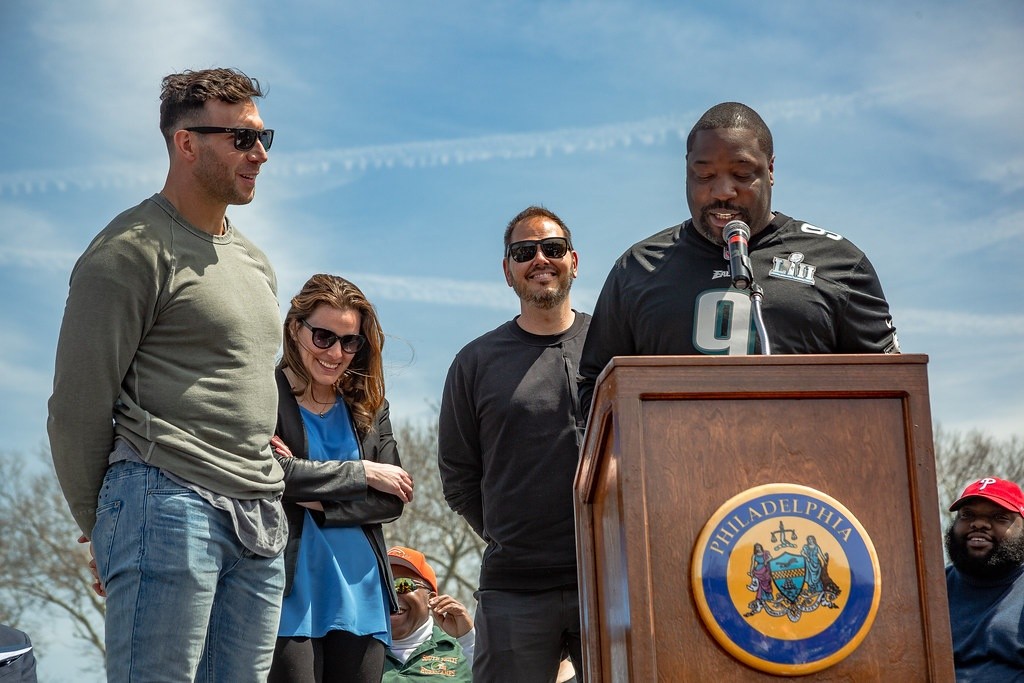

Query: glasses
left=183, top=126, right=274, bottom=152
left=301, top=319, right=367, bottom=354
left=392, top=577, right=433, bottom=594
left=507, top=237, right=572, bottom=263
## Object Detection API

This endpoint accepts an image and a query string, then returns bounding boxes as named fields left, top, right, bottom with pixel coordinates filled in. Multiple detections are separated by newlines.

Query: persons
left=47, top=68, right=289, bottom=683
left=439, top=204, right=592, bottom=683
left=268, top=273, right=415, bottom=683
left=576, top=102, right=901, bottom=425
left=946, top=477, right=1024, bottom=683
left=380, top=546, right=475, bottom=683
left=0, top=624, right=37, bottom=683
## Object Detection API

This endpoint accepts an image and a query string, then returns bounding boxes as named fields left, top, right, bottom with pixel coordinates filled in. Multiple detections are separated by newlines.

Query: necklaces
left=286, top=368, right=331, bottom=418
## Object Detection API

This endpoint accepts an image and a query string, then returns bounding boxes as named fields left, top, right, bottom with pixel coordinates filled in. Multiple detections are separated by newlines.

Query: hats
left=949, top=476, right=1024, bottom=518
left=387, top=546, right=437, bottom=594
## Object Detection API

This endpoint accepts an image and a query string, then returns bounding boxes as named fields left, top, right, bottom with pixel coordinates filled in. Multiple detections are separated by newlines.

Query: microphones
left=722, top=220, right=752, bottom=289
